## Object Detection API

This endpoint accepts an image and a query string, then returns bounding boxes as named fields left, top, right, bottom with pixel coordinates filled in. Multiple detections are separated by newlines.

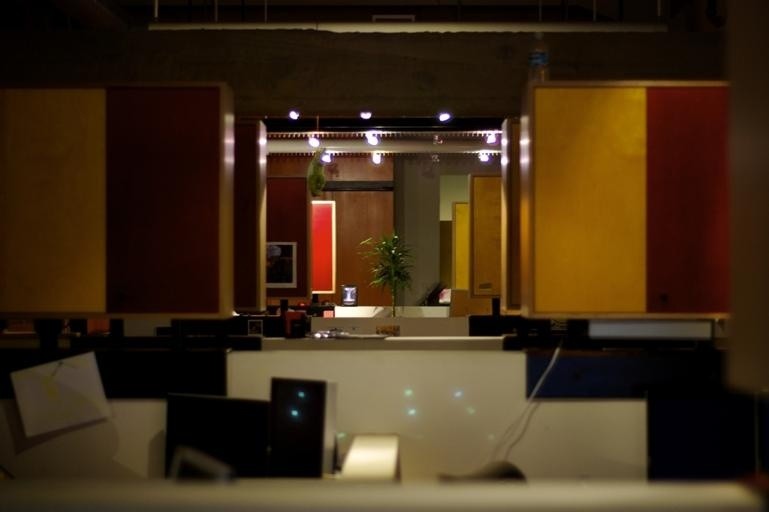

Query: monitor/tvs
left=164, top=392, right=271, bottom=480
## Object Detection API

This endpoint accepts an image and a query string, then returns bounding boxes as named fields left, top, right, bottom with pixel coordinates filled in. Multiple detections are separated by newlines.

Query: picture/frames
left=312, top=200, right=336, bottom=295
left=266, top=242, right=297, bottom=289
